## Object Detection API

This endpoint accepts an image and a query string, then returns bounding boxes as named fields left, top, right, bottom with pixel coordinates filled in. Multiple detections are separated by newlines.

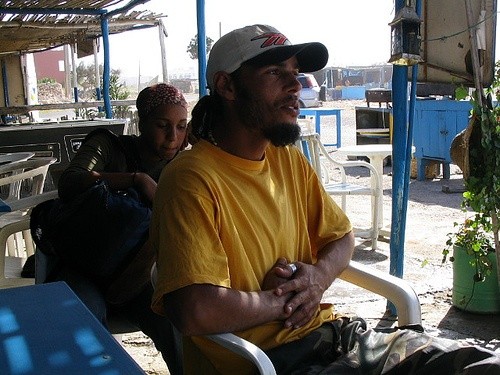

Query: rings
left=286, top=263, right=297, bottom=273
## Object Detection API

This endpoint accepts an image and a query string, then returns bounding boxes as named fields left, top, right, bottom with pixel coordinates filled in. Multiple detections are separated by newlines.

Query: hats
left=136, top=83, right=187, bottom=121
left=205, top=24, right=329, bottom=95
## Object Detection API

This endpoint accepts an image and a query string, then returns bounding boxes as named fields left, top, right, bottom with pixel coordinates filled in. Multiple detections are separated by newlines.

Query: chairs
left=150, top=258, right=422, bottom=375
left=0, top=151, right=58, bottom=288
left=294, top=118, right=378, bottom=251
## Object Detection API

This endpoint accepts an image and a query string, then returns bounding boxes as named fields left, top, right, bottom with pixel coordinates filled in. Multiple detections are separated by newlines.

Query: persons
left=151, top=25, right=500, bottom=375
left=59, top=83, right=188, bottom=375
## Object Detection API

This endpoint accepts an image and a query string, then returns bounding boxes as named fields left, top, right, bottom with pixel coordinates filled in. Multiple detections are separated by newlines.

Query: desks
left=299, top=108, right=342, bottom=148
left=337, top=144, right=416, bottom=238
left=0, top=281, right=147, bottom=375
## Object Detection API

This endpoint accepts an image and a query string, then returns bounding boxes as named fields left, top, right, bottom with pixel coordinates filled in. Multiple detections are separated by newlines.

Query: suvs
left=296, top=73, right=328, bottom=108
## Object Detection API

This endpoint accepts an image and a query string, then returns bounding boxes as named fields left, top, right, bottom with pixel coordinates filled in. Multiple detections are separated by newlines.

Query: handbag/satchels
left=29, top=180, right=155, bottom=293
